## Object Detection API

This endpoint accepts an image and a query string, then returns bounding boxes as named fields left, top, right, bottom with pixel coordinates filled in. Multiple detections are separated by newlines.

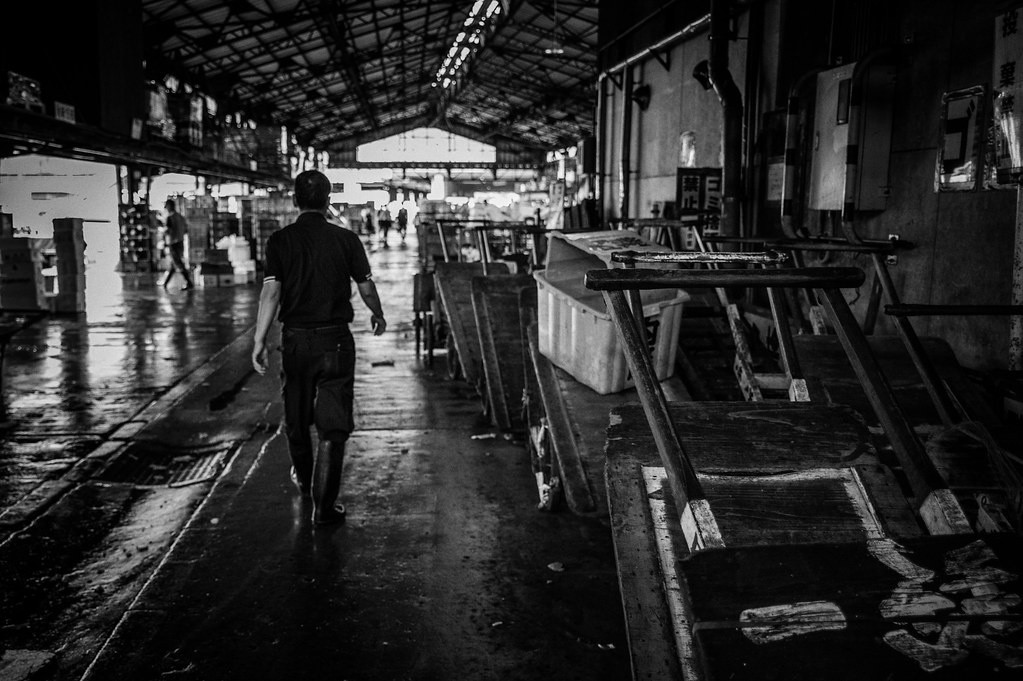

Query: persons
left=162, top=200, right=194, bottom=291
left=252, top=170, right=386, bottom=528
left=362, top=202, right=408, bottom=242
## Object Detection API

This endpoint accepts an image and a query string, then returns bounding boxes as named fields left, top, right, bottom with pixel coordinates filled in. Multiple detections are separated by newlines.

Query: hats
left=164, top=199, right=175, bottom=208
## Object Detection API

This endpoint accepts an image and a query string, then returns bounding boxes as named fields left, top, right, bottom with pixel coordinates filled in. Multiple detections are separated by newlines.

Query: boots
left=284, top=422, right=314, bottom=497
left=156, top=268, right=176, bottom=289
left=313, top=438, right=346, bottom=525
left=180, top=269, right=193, bottom=290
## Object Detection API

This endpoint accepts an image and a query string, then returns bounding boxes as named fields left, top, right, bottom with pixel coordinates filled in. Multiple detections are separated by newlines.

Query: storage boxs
left=0, top=213, right=87, bottom=313
left=543, top=224, right=679, bottom=315
left=202, top=243, right=256, bottom=285
left=532, top=267, right=690, bottom=395
